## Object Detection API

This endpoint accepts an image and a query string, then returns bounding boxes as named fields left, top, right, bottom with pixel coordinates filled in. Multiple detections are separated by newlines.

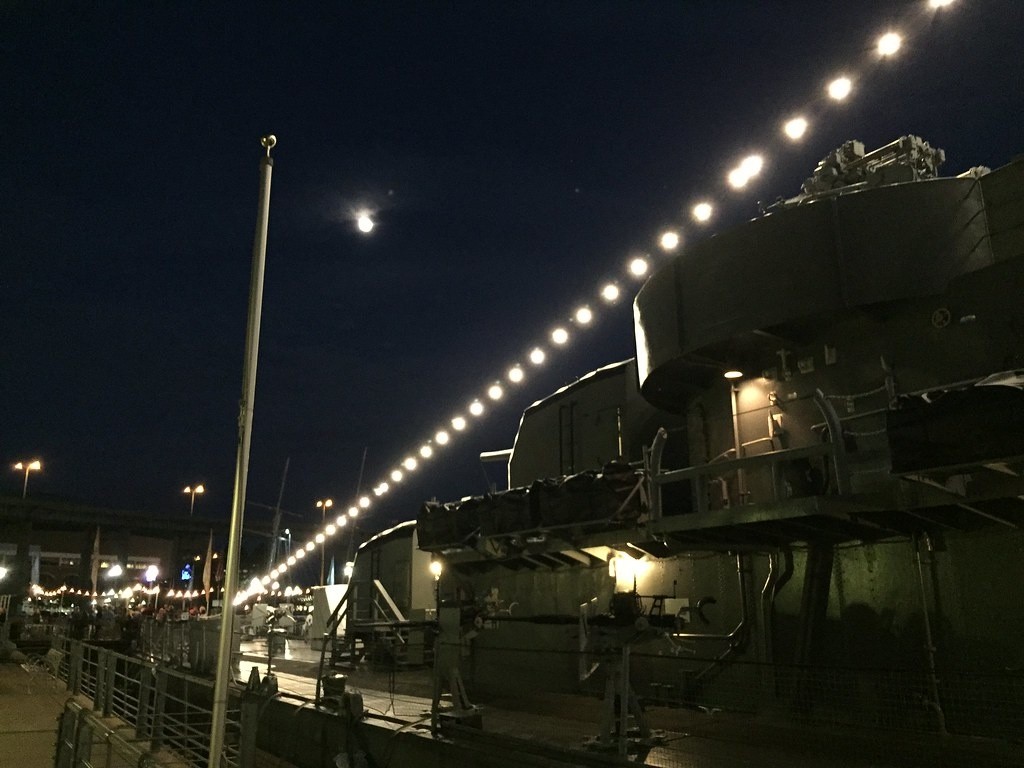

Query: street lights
left=315, top=499, right=334, bottom=588
left=145, top=565, right=160, bottom=608
left=183, top=484, right=204, bottom=515
left=13, top=461, right=41, bottom=499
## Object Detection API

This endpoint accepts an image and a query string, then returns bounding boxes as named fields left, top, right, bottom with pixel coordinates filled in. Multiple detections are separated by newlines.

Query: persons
left=88, top=601, right=206, bottom=632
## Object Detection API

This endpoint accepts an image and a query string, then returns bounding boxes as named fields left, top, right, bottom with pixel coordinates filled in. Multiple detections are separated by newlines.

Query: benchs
left=20, top=648, right=65, bottom=696
